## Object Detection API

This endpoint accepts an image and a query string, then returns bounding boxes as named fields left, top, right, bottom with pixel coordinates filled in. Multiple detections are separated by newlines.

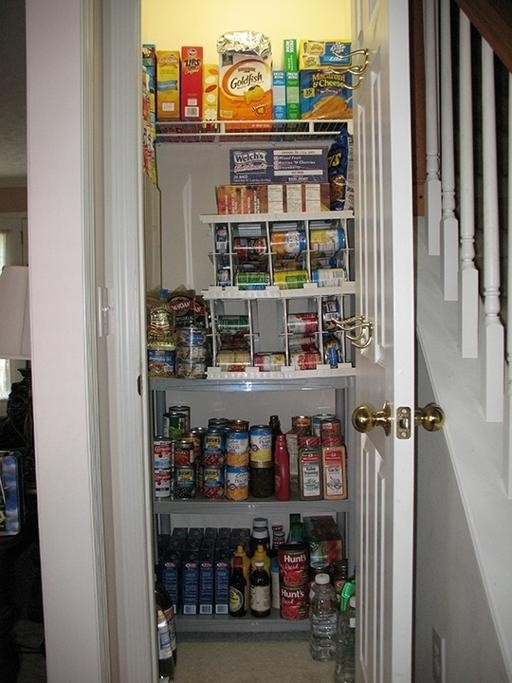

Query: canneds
left=279, top=582, right=310, bottom=621
left=279, top=543, right=311, bottom=587
left=211, top=226, right=346, bottom=291
left=146, top=291, right=344, bottom=382
left=150, top=405, right=347, bottom=502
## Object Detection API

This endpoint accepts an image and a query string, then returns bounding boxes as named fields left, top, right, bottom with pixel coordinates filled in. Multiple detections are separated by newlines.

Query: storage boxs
left=214, top=182, right=332, bottom=216
left=227, top=146, right=331, bottom=187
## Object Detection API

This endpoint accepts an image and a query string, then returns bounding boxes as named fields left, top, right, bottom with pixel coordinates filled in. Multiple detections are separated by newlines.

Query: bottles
left=267, top=415, right=349, bottom=500
left=154, top=566, right=181, bottom=682
left=229, top=509, right=355, bottom=682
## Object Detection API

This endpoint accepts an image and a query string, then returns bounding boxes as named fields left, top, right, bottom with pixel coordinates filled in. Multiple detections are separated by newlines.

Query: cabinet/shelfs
left=193, top=209, right=358, bottom=386
left=144, top=119, right=359, bottom=639
